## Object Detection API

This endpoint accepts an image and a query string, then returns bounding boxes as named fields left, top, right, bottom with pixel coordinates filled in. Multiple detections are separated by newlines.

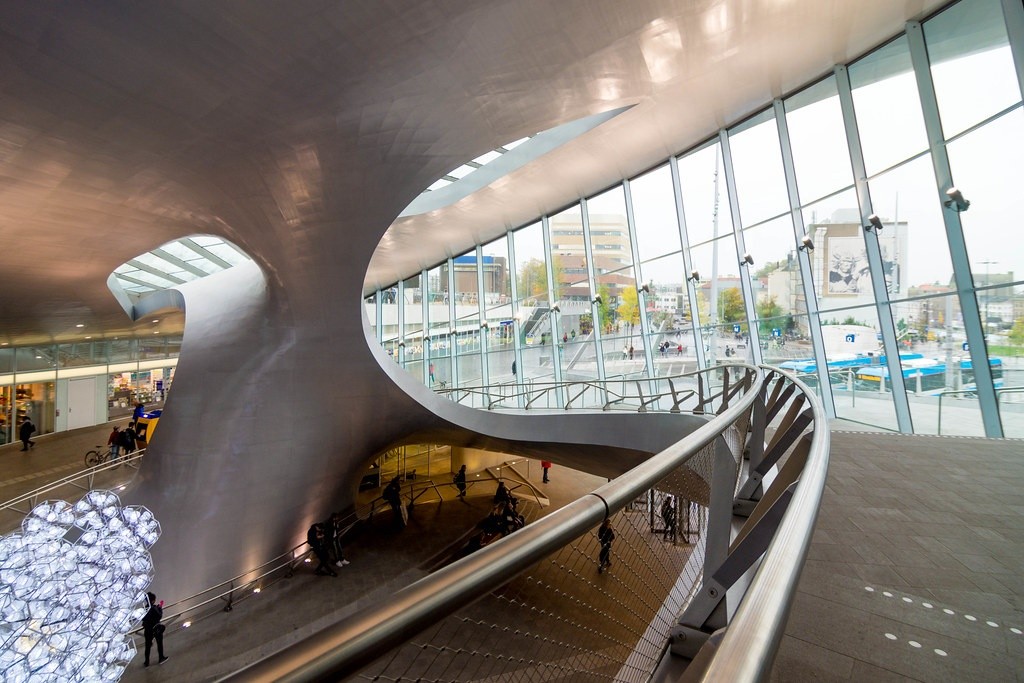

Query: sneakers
left=144, top=662, right=150, bottom=669
left=20, top=447, right=29, bottom=452
left=315, top=570, right=321, bottom=575
left=330, top=572, right=337, bottom=577
left=341, top=559, right=350, bottom=565
left=30, top=442, right=37, bottom=448
left=336, top=561, right=342, bottom=567
left=546, top=479, right=550, bottom=481
left=159, top=657, right=170, bottom=665
left=543, top=480, right=547, bottom=483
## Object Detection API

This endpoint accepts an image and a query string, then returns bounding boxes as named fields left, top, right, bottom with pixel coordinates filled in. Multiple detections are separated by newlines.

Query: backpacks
left=453, top=472, right=460, bottom=485
left=383, top=484, right=392, bottom=500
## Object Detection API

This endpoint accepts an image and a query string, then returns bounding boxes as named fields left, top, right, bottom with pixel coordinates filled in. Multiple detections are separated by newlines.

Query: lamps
left=800, top=234, right=814, bottom=254
left=739, top=252, right=756, bottom=273
left=864, top=212, right=882, bottom=234
left=392, top=266, right=699, bottom=348
left=944, top=186, right=969, bottom=213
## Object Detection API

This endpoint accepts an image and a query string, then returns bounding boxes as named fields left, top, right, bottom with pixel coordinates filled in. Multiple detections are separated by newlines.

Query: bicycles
left=84, top=445, right=123, bottom=471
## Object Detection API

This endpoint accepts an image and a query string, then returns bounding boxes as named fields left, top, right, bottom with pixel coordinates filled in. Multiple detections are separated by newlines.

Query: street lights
left=978, top=261, right=1000, bottom=339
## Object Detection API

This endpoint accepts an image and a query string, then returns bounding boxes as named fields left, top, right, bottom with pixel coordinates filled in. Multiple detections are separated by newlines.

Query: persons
left=487, top=480, right=524, bottom=536
left=107, top=426, right=122, bottom=466
left=598, top=519, right=615, bottom=573
left=540, top=460, right=551, bottom=483
left=18, top=416, right=36, bottom=452
left=383, top=475, right=405, bottom=526
left=453, top=464, right=467, bottom=499
left=119, top=421, right=142, bottom=462
left=131, top=401, right=145, bottom=430
left=321, top=514, right=351, bottom=568
left=661, top=496, right=675, bottom=540
left=307, top=522, right=338, bottom=579
left=421, top=320, right=787, bottom=385
left=140, top=590, right=170, bottom=669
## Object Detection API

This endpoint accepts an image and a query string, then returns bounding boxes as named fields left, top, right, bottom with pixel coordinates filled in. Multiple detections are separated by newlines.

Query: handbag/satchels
left=152, top=624, right=166, bottom=638
left=26, top=424, right=36, bottom=434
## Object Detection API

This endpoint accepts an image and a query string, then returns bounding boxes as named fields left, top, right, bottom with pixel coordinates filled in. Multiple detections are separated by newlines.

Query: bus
left=854, top=354, right=1004, bottom=401
left=778, top=351, right=924, bottom=391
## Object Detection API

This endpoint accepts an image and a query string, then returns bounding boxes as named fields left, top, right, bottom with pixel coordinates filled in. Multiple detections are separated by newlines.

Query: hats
left=113, top=426, right=121, bottom=430
